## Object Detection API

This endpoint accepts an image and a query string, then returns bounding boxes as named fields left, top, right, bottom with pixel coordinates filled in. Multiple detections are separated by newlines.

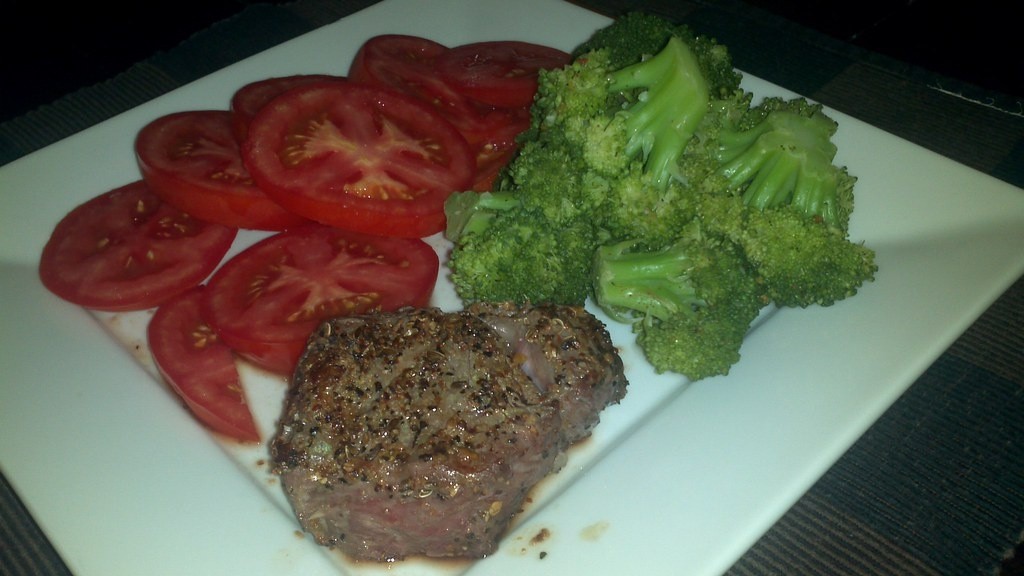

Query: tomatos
left=37, top=35, right=577, bottom=442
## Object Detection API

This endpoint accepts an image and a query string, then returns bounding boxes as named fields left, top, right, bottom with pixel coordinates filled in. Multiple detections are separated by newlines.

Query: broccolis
left=443, top=11, right=878, bottom=379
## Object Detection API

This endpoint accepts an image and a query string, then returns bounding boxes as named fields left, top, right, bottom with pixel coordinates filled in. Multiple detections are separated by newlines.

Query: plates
left=0, top=0, right=1024, bottom=576
